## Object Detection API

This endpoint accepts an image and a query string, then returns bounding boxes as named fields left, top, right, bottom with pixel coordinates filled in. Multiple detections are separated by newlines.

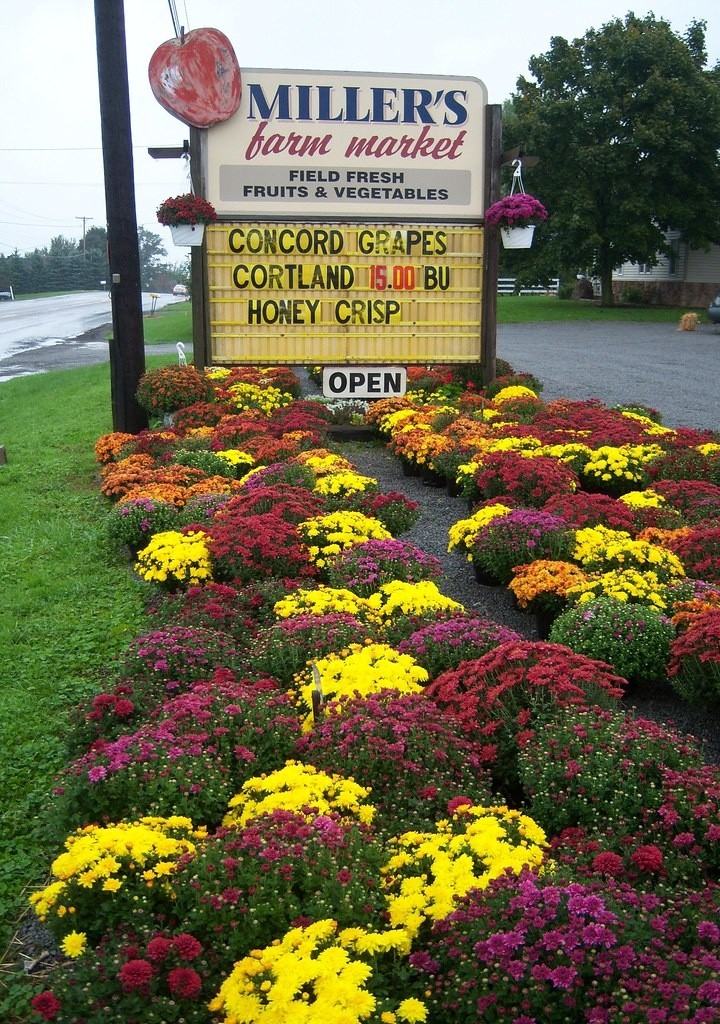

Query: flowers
left=20, top=353, right=718, bottom=1024
left=154, top=194, right=219, bottom=229
left=485, top=193, right=545, bottom=234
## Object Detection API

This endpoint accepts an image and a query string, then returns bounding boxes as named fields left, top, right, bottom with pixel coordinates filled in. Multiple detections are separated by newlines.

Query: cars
left=172, top=285, right=188, bottom=297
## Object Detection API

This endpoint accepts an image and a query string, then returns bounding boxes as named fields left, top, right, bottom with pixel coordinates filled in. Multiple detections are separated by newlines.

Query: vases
left=501, top=225, right=537, bottom=248
left=168, top=222, right=206, bottom=247
